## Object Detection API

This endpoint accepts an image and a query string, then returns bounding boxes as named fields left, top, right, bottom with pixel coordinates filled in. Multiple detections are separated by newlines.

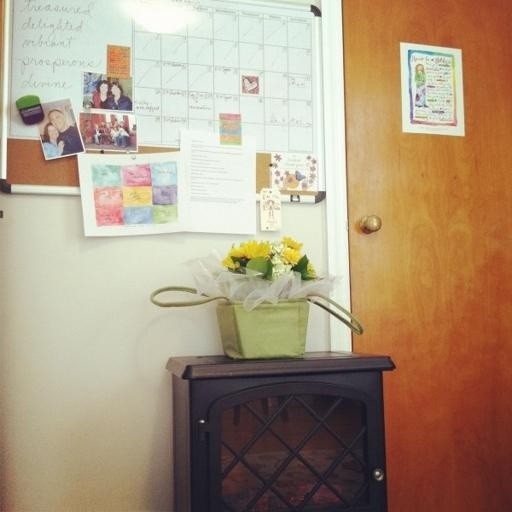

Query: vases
left=213, top=294, right=311, bottom=360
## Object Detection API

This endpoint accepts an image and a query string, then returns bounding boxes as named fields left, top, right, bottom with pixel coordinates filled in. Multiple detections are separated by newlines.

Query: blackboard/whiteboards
left=2, top=0, right=326, bottom=204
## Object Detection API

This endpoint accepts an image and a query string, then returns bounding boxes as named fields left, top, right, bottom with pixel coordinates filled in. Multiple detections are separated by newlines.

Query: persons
left=42, top=80, right=136, bottom=158
left=415, top=64, right=427, bottom=107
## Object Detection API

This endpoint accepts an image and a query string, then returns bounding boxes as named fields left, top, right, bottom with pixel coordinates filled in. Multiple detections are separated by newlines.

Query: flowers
left=216, top=231, right=321, bottom=288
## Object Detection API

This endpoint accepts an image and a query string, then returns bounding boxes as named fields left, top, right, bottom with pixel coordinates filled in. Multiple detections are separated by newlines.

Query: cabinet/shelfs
left=164, top=351, right=398, bottom=512
